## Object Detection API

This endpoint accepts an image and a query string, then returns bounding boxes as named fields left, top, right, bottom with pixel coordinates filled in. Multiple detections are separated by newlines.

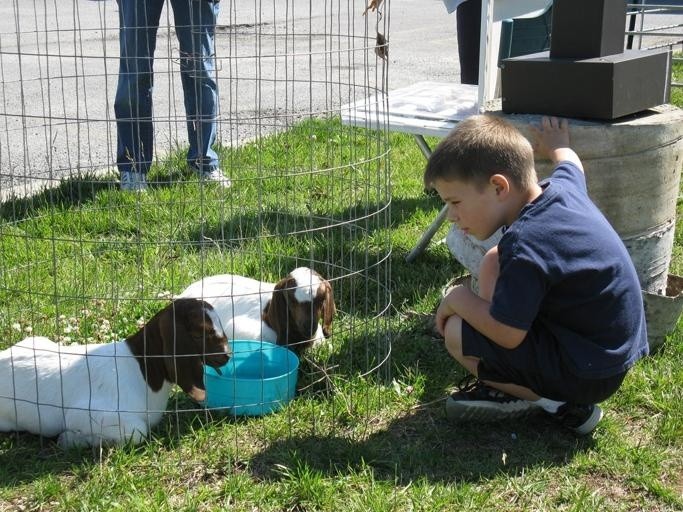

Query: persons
left=113, top=0, right=234, bottom=194
left=423, top=111, right=652, bottom=437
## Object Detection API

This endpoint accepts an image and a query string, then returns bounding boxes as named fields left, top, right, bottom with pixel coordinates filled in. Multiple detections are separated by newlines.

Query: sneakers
left=119, top=171, right=151, bottom=193
left=188, top=164, right=231, bottom=190
left=444, top=380, right=544, bottom=426
left=541, top=401, right=603, bottom=437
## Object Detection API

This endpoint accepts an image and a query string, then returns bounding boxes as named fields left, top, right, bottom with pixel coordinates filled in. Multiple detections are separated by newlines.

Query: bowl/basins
left=196, top=340, right=300, bottom=418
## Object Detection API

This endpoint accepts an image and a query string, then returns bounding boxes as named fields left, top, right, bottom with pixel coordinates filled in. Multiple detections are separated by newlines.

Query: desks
left=339, top=80, right=481, bottom=257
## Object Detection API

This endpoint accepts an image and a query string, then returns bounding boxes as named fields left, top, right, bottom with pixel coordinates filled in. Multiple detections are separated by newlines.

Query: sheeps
left=177, top=263, right=337, bottom=350
left=0, top=296, right=231, bottom=453
